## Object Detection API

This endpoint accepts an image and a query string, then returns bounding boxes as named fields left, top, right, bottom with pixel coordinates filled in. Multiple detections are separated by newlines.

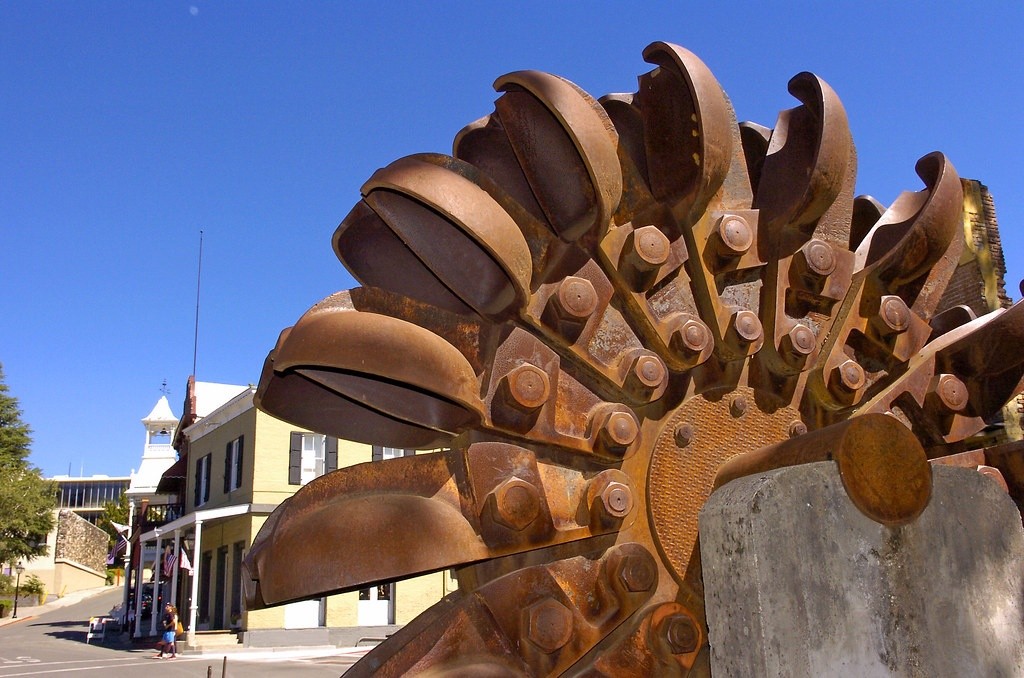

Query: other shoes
left=169, top=657, right=176, bottom=660
left=152, top=657, right=162, bottom=659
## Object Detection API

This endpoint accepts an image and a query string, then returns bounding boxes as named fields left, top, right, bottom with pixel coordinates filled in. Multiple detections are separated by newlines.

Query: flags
left=109, top=521, right=130, bottom=560
left=180, top=548, right=191, bottom=571
left=163, top=553, right=177, bottom=577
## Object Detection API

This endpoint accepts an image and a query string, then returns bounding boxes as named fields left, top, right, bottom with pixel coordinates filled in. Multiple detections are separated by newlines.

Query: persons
left=152, top=603, right=178, bottom=659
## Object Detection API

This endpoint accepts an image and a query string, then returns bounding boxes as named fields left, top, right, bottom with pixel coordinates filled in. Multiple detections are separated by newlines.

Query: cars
left=129, top=581, right=164, bottom=619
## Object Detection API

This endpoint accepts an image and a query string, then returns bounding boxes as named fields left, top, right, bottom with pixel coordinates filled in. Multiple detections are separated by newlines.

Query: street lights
left=12, top=561, right=25, bottom=618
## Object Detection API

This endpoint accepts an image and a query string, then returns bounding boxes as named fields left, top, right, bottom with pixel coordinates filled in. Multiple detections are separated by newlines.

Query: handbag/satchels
left=175, top=622, right=183, bottom=636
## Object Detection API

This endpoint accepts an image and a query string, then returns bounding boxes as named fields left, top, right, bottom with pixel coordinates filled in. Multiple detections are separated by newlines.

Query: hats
left=165, top=602, right=171, bottom=605
